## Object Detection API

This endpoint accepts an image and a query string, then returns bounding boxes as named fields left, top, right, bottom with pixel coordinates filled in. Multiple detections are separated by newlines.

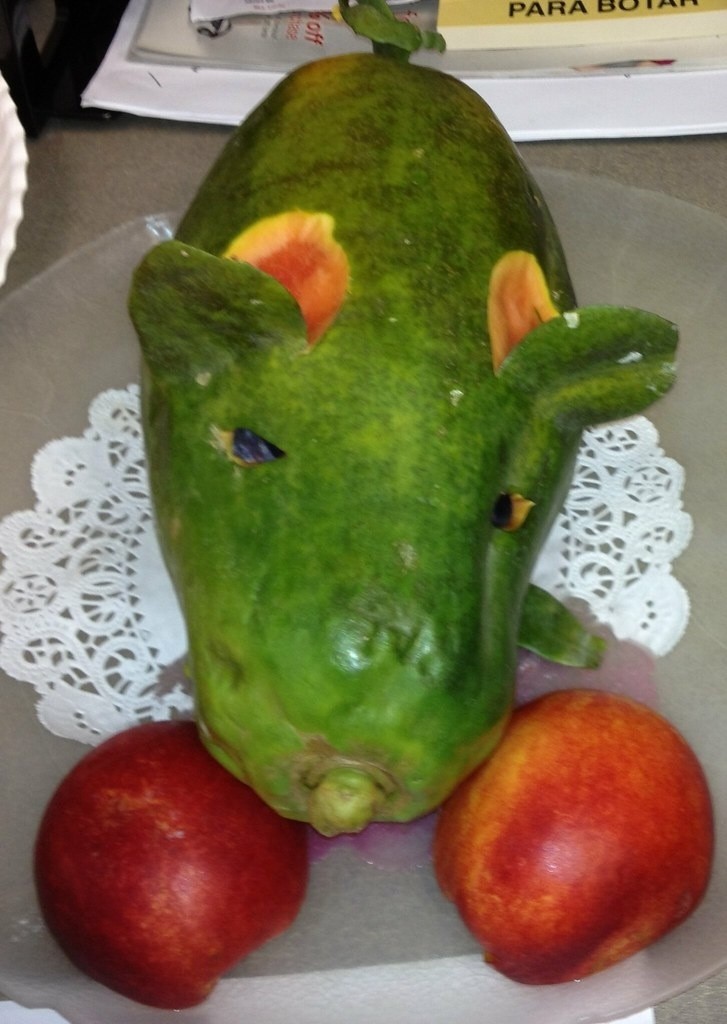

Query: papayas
left=125, top=52, right=680, bottom=837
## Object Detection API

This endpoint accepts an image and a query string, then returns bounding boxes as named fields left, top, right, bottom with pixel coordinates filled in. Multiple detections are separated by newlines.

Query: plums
left=34, top=717, right=312, bottom=1009
left=431, top=688, right=714, bottom=986
